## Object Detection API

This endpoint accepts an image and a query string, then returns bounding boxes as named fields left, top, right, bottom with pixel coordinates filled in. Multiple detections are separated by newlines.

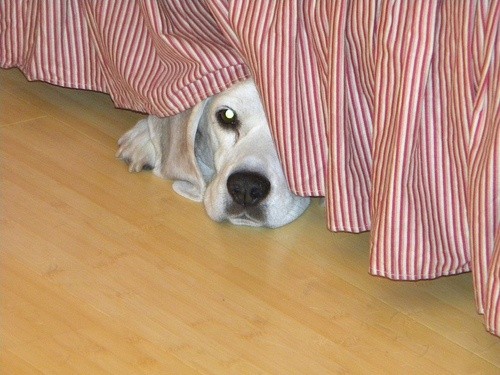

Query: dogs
left=117, top=77, right=311, bottom=228
